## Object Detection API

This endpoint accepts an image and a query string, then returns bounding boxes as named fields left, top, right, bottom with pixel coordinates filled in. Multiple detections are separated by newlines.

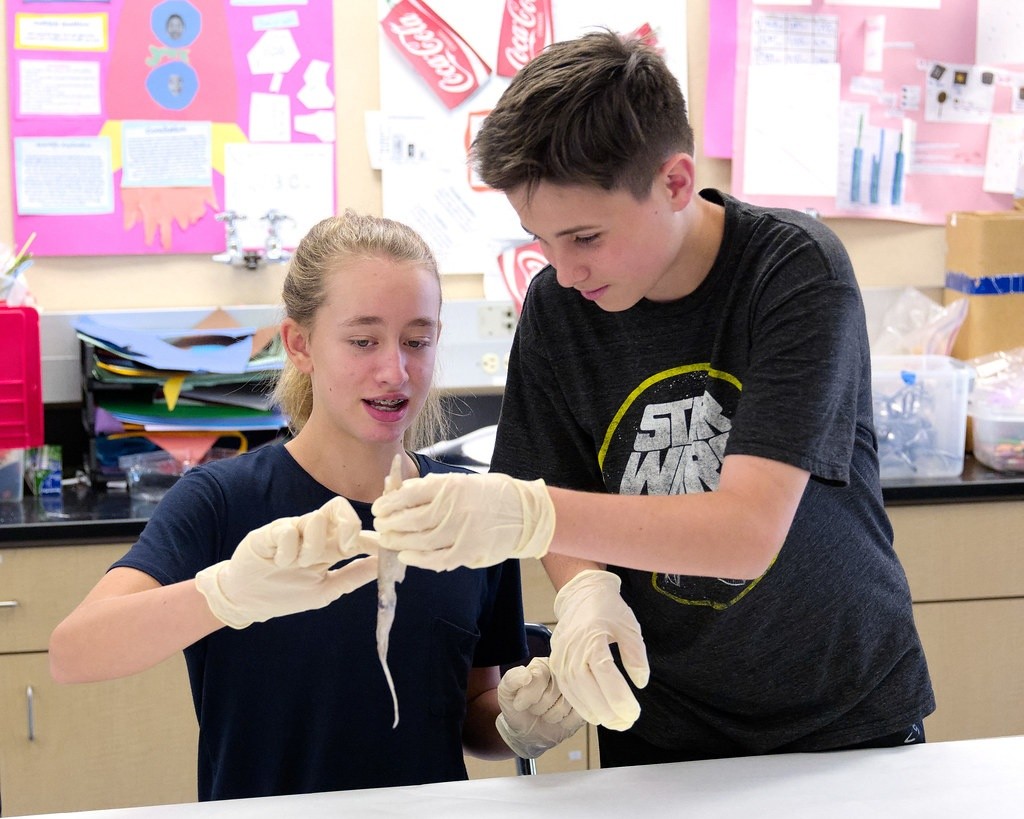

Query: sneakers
left=496, top=657, right=587, bottom=759
left=548, top=570, right=650, bottom=732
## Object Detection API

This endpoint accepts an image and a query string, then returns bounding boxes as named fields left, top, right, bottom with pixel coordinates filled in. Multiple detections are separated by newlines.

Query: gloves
left=195, top=498, right=381, bottom=629
left=372, top=474, right=555, bottom=573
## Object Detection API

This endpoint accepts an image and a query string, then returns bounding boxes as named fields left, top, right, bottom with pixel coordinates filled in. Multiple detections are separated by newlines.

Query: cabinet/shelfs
left=0, top=549, right=588, bottom=818
left=886, top=503, right=1024, bottom=743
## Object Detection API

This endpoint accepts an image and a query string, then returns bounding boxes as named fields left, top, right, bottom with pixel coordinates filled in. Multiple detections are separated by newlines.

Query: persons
left=45, top=210, right=582, bottom=809
left=367, top=29, right=937, bottom=773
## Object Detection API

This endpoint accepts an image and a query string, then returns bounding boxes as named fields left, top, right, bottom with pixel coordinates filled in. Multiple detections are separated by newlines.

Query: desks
left=46, top=734, right=1024, bottom=817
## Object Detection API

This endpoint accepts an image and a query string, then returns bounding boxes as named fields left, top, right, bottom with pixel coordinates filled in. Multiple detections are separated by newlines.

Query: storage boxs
left=869, top=353, right=976, bottom=480
left=944, top=209, right=1023, bottom=454
left=965, top=354, right=1024, bottom=473
